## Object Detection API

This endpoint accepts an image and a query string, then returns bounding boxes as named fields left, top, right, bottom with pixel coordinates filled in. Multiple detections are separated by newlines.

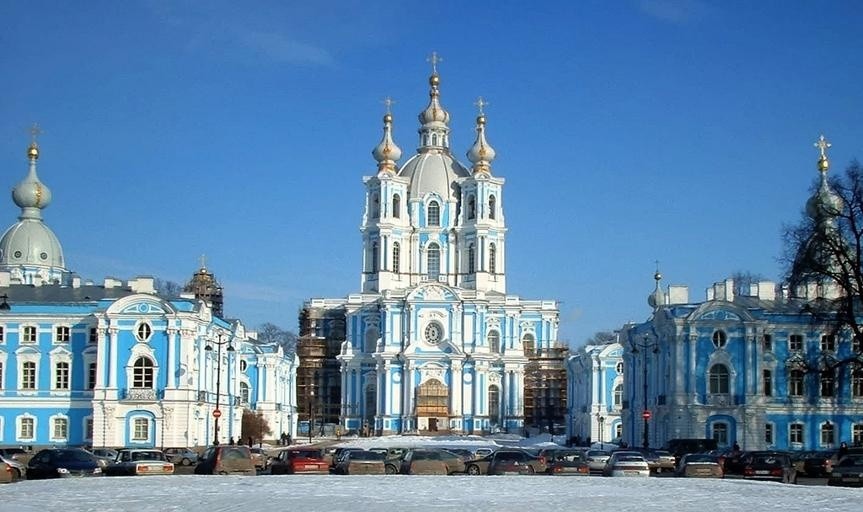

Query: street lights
left=205, top=328, right=236, bottom=447
left=631, top=336, right=661, bottom=447
left=308, top=386, right=317, bottom=443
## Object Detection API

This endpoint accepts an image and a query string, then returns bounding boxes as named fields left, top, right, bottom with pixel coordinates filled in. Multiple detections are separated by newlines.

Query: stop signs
left=212, top=410, right=221, bottom=418
left=642, top=411, right=651, bottom=419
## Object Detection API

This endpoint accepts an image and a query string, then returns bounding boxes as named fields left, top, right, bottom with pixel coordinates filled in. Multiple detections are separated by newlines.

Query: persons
left=617, top=438, right=631, bottom=448
left=837, top=441, right=851, bottom=461
left=281, top=430, right=285, bottom=445
left=248, top=437, right=252, bottom=447
left=285, top=432, right=292, bottom=445
left=230, top=436, right=234, bottom=444
left=567, top=433, right=591, bottom=448
left=732, top=440, right=740, bottom=452
left=238, top=439, right=242, bottom=445
